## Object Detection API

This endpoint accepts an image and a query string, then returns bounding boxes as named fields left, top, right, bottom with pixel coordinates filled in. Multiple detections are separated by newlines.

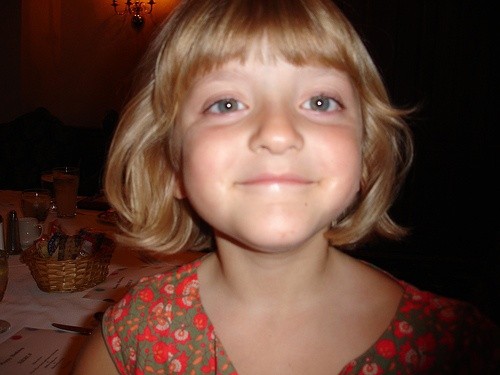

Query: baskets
left=20, top=220, right=117, bottom=294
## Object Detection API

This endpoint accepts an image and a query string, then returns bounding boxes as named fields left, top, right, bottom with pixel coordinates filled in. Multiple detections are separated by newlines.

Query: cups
left=41, top=168, right=55, bottom=198
left=53, top=166, right=80, bottom=198
left=18, top=217, right=44, bottom=252
left=22, top=188, right=50, bottom=217
left=53, top=170, right=74, bottom=218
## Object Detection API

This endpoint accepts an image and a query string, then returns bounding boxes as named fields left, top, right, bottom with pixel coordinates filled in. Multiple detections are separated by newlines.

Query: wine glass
left=0, top=255, right=10, bottom=333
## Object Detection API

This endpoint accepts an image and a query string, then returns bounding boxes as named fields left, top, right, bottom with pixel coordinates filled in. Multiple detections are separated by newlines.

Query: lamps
left=112, top=0, right=155, bottom=32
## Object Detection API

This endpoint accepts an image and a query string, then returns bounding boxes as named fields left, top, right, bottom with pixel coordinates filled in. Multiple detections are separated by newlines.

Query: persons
left=72, top=0, right=500, bottom=375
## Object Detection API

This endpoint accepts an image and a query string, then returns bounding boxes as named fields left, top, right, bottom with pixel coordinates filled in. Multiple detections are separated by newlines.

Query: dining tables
left=0, top=189, right=211, bottom=375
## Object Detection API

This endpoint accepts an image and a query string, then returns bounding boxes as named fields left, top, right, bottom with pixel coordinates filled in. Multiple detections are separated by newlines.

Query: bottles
left=0, top=215, right=5, bottom=252
left=6, top=210, right=22, bottom=254
